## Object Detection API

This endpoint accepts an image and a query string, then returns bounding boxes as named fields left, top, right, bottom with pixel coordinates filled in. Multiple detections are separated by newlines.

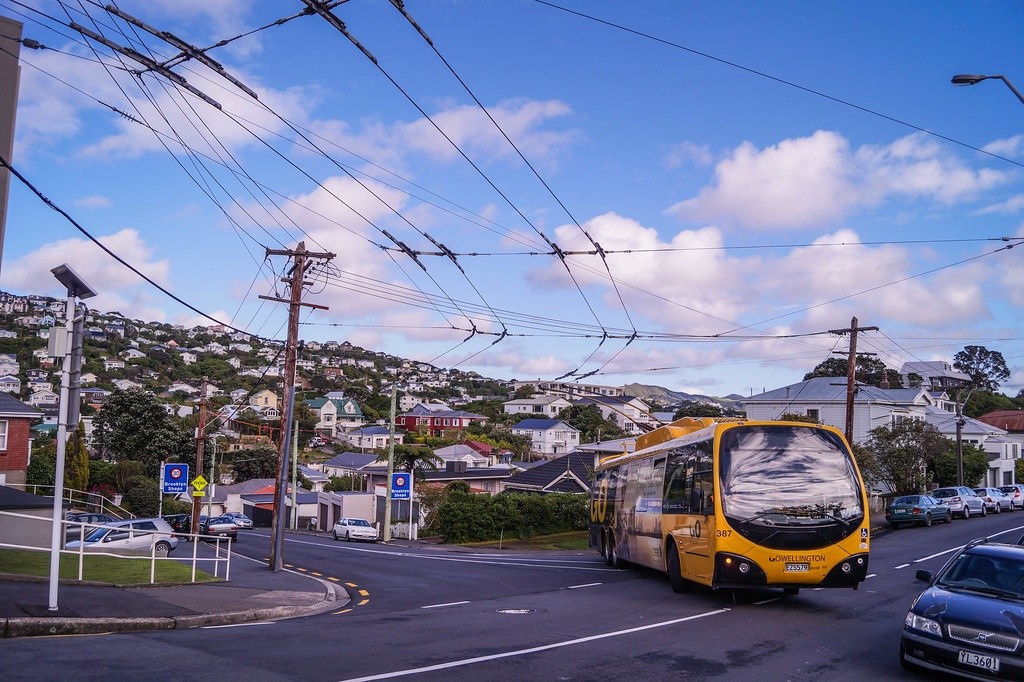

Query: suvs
left=997, top=484, right=1023, bottom=510
left=931, top=486, right=987, bottom=519
left=63, top=515, right=178, bottom=559
left=162, top=514, right=190, bottom=535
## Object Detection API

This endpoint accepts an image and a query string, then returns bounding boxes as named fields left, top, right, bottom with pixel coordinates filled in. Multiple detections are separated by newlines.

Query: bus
left=588, top=416, right=869, bottom=599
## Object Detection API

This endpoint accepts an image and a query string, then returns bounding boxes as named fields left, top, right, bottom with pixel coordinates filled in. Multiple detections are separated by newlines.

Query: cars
left=331, top=517, right=379, bottom=542
left=182, top=515, right=210, bottom=540
left=884, top=494, right=953, bottom=528
left=65, top=513, right=123, bottom=542
left=307, top=437, right=333, bottom=448
left=972, top=488, right=1015, bottom=514
left=204, top=517, right=240, bottom=544
left=897, top=538, right=1024, bottom=682
left=220, top=512, right=254, bottom=529
